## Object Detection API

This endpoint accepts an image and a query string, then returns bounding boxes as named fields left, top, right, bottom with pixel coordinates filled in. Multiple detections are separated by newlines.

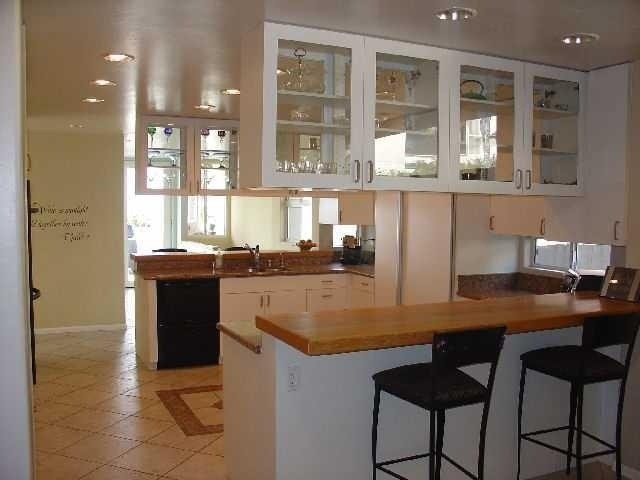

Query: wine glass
left=217, top=129, right=227, bottom=147
left=200, top=129, right=210, bottom=148
left=164, top=127, right=173, bottom=145
left=148, top=127, right=156, bottom=148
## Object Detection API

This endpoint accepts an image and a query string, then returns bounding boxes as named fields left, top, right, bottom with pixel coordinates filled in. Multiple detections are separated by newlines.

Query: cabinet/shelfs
left=135, top=249, right=374, bottom=372
left=136, top=112, right=342, bottom=201
left=486, top=61, right=633, bottom=247
left=446, top=46, right=585, bottom=200
left=236, top=23, right=447, bottom=197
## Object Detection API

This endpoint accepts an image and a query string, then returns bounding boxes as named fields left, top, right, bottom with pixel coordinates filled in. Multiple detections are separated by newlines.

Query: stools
left=369, top=326, right=505, bottom=480
left=515, top=310, right=638, bottom=480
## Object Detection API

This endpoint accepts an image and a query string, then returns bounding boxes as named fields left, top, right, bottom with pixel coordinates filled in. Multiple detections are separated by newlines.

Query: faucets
left=242, top=243, right=260, bottom=267
left=280, top=250, right=285, bottom=266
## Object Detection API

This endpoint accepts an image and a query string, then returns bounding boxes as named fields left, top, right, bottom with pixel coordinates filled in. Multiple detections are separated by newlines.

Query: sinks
left=257, top=266, right=290, bottom=274
left=216, top=268, right=257, bottom=275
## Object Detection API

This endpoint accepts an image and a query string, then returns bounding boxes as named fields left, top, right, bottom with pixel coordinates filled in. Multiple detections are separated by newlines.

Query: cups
left=296, top=111, right=305, bottom=121
